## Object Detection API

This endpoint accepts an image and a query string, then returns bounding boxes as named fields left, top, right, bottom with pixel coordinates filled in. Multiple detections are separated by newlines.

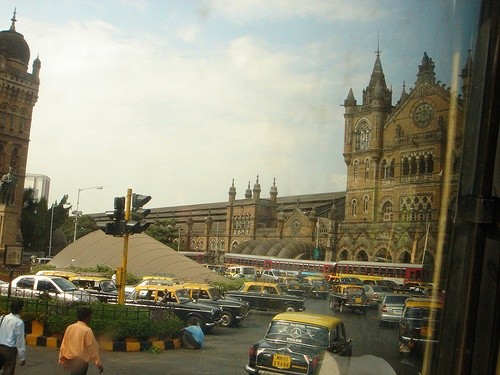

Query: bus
left=335, top=261, right=424, bottom=282
left=224, top=254, right=337, bottom=275
left=177, top=252, right=207, bottom=264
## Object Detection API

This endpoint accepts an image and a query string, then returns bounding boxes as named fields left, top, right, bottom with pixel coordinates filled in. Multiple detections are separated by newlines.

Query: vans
left=225, top=266, right=256, bottom=281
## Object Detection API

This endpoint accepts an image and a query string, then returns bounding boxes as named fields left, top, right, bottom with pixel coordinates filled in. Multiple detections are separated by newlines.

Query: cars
left=275, top=277, right=305, bottom=295
left=204, top=265, right=225, bottom=274
left=170, top=282, right=249, bottom=326
left=261, top=268, right=434, bottom=308
left=224, top=282, right=306, bottom=314
left=35, top=270, right=182, bottom=304
left=108, top=285, right=230, bottom=336
left=398, top=301, right=442, bottom=355
left=0, top=275, right=99, bottom=303
left=329, top=284, right=370, bottom=314
left=376, top=294, right=412, bottom=327
left=245, top=311, right=353, bottom=375
left=303, top=277, right=330, bottom=299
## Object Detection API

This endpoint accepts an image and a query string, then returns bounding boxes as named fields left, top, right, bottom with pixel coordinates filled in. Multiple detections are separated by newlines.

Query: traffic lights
left=123, top=193, right=153, bottom=235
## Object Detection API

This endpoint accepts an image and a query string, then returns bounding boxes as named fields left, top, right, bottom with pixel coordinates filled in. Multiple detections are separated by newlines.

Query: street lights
left=47, top=201, right=72, bottom=258
left=177, top=228, right=183, bottom=252
left=74, top=185, right=103, bottom=241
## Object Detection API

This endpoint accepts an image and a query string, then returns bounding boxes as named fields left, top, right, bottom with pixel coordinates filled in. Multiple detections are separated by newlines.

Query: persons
left=111, top=270, right=117, bottom=285
left=0, top=297, right=26, bottom=375
left=181, top=319, right=204, bottom=349
left=58, top=307, right=104, bottom=375
left=0, top=166, right=18, bottom=206
